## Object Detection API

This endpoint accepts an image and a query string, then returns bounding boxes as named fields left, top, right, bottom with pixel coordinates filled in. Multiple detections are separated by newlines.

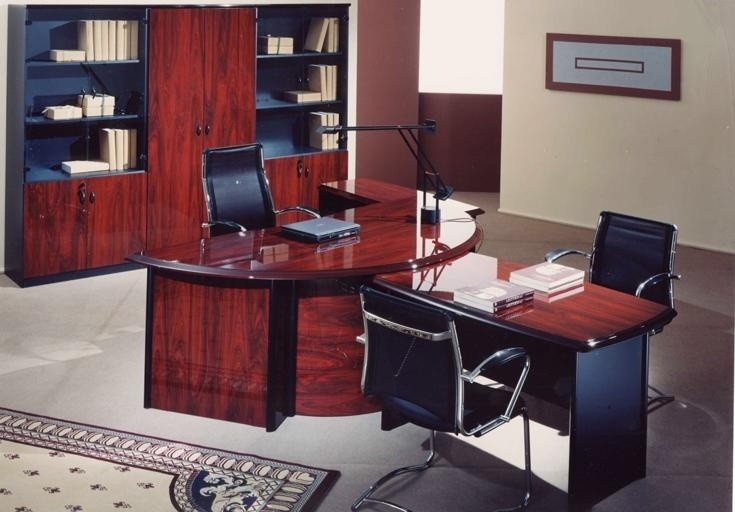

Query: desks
left=372, top=252, right=679, bottom=509
left=128, top=174, right=485, bottom=432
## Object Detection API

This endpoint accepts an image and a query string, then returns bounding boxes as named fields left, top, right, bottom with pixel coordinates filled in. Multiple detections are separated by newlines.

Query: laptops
left=280, top=217, right=361, bottom=243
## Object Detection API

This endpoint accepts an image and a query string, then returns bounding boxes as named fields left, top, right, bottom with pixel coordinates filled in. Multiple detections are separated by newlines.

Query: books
left=487, top=301, right=534, bottom=320
left=44, top=18, right=140, bottom=176
left=453, top=277, right=535, bottom=307
left=453, top=296, right=534, bottom=314
left=256, top=16, right=341, bottom=150
left=510, top=262, right=585, bottom=289
left=533, top=285, right=584, bottom=303
left=511, top=278, right=583, bottom=294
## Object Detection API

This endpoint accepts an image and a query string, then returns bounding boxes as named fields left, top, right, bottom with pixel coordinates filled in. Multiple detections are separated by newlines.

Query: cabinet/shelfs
left=256, top=3, right=349, bottom=226
left=145, top=7, right=255, bottom=249
left=3, top=4, right=146, bottom=285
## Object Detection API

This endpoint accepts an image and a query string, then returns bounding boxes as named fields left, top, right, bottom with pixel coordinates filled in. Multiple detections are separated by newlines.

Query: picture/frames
left=546, top=30, right=682, bottom=104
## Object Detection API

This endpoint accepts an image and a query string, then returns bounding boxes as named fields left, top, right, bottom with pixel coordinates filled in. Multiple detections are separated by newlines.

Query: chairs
left=344, top=284, right=536, bottom=512
left=543, top=209, right=678, bottom=418
left=201, top=143, right=322, bottom=240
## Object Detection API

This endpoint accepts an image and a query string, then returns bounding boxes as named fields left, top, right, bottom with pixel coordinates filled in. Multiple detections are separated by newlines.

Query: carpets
left=0, top=406, right=342, bottom=511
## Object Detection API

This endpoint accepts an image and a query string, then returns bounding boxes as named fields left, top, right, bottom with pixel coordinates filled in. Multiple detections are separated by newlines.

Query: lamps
left=316, top=117, right=456, bottom=226
